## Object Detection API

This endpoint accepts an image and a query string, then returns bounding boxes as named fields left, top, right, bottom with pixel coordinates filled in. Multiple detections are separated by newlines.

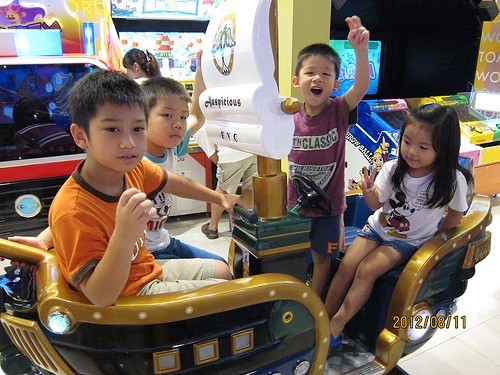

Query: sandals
left=201, top=223, right=218, bottom=239
left=329, top=333, right=345, bottom=348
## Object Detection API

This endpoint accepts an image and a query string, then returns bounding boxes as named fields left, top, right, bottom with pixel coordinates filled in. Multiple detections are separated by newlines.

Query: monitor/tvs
left=377, top=111, right=408, bottom=129
left=329, top=36, right=386, bottom=100
left=443, top=104, right=487, bottom=122
left=118, top=31, right=205, bottom=81
left=0, top=63, right=95, bottom=162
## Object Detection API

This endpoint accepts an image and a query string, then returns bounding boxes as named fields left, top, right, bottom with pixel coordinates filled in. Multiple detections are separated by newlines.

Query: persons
left=139, top=50, right=235, bottom=280
left=324, top=103, right=469, bottom=348
left=13, top=98, right=77, bottom=155
left=122, top=48, right=163, bottom=84
left=48, top=69, right=244, bottom=307
left=187, top=114, right=259, bottom=239
left=287, top=16, right=370, bottom=299
